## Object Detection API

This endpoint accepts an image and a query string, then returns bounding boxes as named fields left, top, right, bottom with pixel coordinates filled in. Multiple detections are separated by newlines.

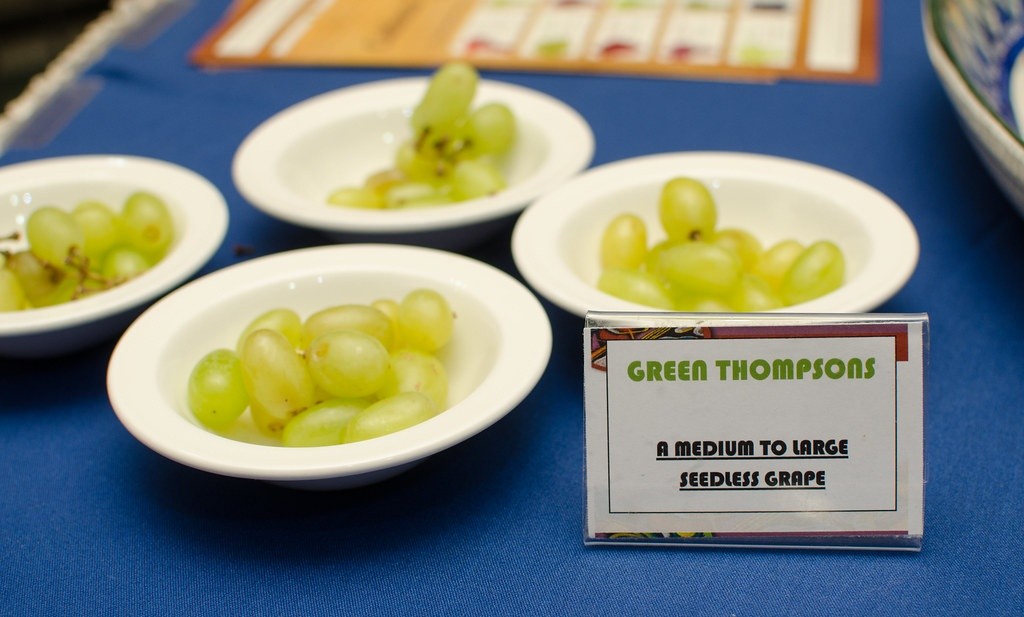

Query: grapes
left=333, top=59, right=516, bottom=208
left=0, top=190, right=171, bottom=313
left=596, top=177, right=845, bottom=314
left=188, top=291, right=455, bottom=447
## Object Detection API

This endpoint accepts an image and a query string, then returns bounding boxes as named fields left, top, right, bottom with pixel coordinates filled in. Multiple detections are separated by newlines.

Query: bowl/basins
left=1, top=156, right=228, bottom=336
left=104, top=243, right=554, bottom=479
left=916, top=1, right=1024, bottom=218
left=228, top=76, right=594, bottom=232
left=509, top=150, right=920, bottom=324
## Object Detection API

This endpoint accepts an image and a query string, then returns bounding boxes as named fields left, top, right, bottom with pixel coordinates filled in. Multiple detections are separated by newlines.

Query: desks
left=0, top=0, right=1024, bottom=617
